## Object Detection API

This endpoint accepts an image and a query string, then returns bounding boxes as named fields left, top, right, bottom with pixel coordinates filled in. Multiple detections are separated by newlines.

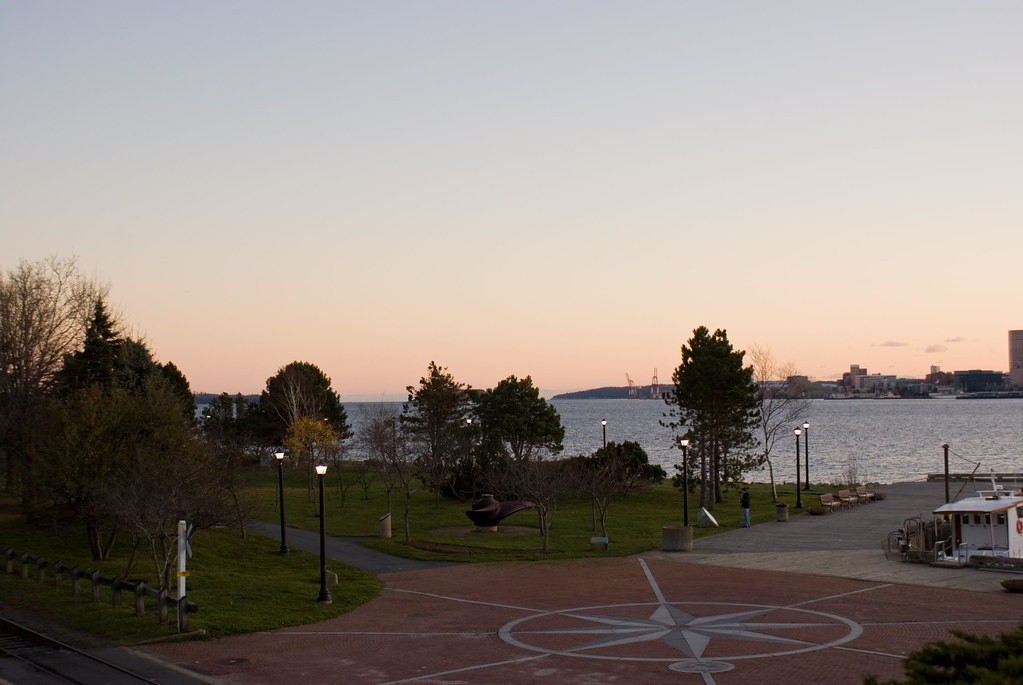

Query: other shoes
left=742, top=526, right=750, bottom=528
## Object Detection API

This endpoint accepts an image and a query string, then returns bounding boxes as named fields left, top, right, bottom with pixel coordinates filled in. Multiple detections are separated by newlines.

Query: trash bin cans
left=776, top=504, right=787, bottom=522
left=378, top=513, right=391, bottom=538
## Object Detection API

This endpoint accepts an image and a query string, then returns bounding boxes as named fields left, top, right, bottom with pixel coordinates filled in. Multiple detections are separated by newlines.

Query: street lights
left=315, top=458, right=330, bottom=602
left=794, top=425, right=803, bottom=508
left=274, top=447, right=290, bottom=555
left=802, top=420, right=812, bottom=491
left=601, top=418, right=606, bottom=449
left=681, top=434, right=690, bottom=527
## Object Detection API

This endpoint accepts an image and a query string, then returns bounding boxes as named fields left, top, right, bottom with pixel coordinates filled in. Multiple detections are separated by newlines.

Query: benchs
left=855, top=485, right=876, bottom=504
left=820, top=493, right=844, bottom=513
left=838, top=490, right=860, bottom=508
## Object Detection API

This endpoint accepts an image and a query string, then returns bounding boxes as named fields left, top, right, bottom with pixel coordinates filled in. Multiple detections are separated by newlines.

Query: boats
left=931, top=464, right=1023, bottom=567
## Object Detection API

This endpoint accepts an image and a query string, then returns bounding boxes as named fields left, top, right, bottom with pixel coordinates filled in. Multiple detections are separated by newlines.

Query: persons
left=740, top=487, right=750, bottom=527
